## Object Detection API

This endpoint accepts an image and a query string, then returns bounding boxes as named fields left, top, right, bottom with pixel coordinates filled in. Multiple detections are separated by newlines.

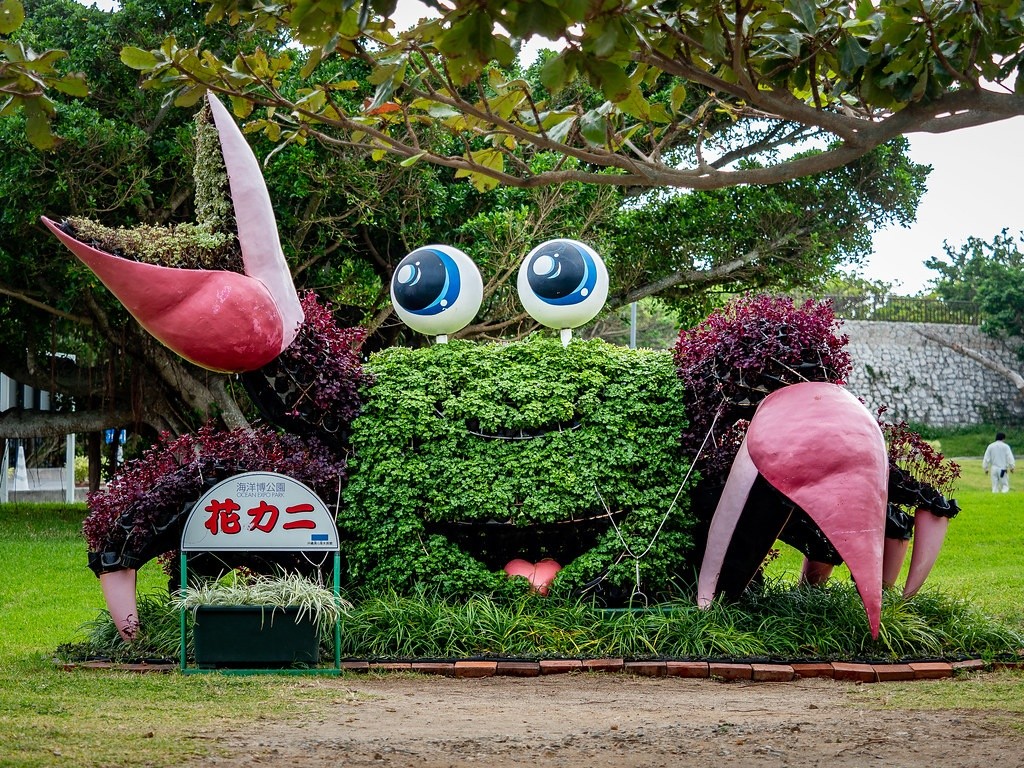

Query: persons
left=982, top=433, right=1015, bottom=493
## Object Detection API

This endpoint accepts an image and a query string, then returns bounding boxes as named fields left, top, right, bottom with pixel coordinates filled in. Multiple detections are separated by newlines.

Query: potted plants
left=163, top=563, right=357, bottom=668
